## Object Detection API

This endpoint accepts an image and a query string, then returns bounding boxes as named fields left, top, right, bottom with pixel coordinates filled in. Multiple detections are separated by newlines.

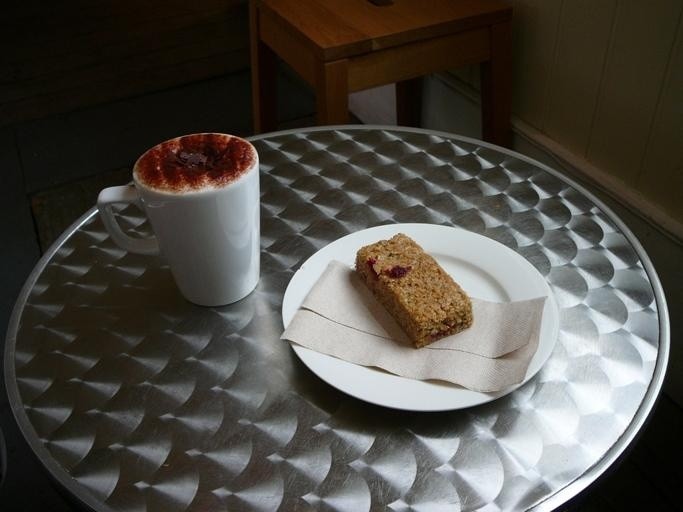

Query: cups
left=95, top=130, right=262, bottom=307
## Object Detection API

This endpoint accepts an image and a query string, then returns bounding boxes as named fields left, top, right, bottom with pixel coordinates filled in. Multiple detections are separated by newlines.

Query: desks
left=249, top=0, right=513, bottom=149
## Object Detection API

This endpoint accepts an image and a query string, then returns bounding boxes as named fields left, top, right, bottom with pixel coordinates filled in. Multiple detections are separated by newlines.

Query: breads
left=353, top=234, right=473, bottom=349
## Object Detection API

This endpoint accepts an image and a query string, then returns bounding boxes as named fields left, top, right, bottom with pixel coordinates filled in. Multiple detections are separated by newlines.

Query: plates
left=280, top=222, right=557, bottom=413
